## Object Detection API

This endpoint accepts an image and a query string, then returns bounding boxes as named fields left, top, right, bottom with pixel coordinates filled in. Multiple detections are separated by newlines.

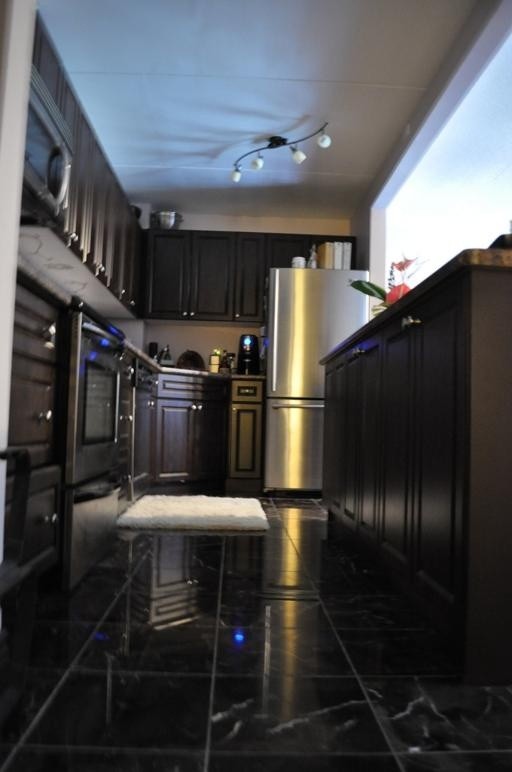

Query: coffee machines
left=237, top=334, right=259, bottom=375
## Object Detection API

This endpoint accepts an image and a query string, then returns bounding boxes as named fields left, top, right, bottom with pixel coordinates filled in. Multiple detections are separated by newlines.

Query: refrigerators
left=263, top=268, right=370, bottom=490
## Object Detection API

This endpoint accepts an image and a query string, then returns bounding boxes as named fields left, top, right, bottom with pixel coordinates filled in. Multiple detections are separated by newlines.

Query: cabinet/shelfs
left=3, top=268, right=268, bottom=640
left=25, top=10, right=357, bottom=327
left=310, top=232, right=512, bottom=646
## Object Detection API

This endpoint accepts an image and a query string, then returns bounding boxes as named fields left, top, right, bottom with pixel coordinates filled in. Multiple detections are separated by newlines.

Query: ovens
left=63, top=305, right=135, bottom=596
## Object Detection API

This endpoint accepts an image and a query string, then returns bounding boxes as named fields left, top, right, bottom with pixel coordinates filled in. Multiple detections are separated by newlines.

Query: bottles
left=307, top=244, right=320, bottom=268
left=218, top=349, right=236, bottom=374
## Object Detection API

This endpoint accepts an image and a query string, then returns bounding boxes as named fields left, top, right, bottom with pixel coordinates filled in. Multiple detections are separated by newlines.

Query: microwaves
left=20, top=86, right=71, bottom=230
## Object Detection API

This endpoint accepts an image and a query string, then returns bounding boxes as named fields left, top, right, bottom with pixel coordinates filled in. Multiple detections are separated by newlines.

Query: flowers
left=350, top=258, right=416, bottom=317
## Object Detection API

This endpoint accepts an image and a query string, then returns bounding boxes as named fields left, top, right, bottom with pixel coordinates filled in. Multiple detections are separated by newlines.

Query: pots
left=150, top=209, right=184, bottom=230
